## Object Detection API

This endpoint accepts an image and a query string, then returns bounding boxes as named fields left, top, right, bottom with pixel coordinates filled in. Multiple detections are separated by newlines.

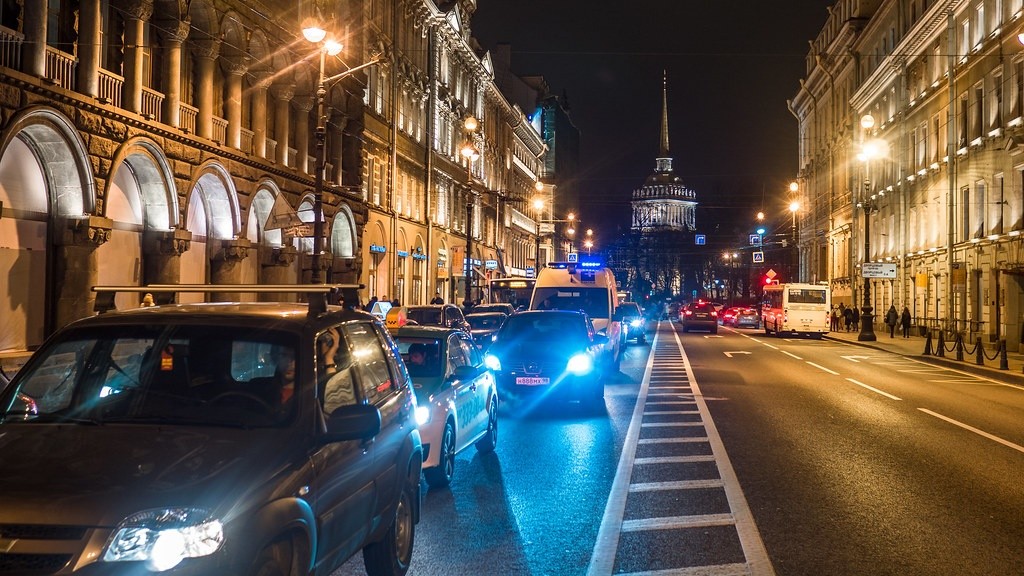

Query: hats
left=834, top=305, right=839, bottom=309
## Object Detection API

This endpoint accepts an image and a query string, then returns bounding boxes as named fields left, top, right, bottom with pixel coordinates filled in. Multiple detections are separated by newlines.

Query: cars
left=617, top=292, right=645, bottom=352
left=385, top=324, right=499, bottom=488
left=481, top=306, right=609, bottom=408
left=665, top=302, right=718, bottom=334
left=474, top=303, right=514, bottom=316
left=713, top=302, right=759, bottom=328
left=405, top=305, right=508, bottom=348
left=100, top=343, right=277, bottom=400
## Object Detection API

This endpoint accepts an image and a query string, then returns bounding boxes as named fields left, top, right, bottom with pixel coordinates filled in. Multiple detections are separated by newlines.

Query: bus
left=761, top=283, right=831, bottom=339
left=480, top=278, right=536, bottom=311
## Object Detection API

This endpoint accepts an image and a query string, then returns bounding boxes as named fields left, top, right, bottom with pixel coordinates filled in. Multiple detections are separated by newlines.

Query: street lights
left=756, top=212, right=766, bottom=272
left=532, top=175, right=544, bottom=279
left=301, top=3, right=343, bottom=283
left=461, top=117, right=480, bottom=316
left=858, top=115, right=876, bottom=341
left=789, top=183, right=797, bottom=282
left=568, top=213, right=593, bottom=256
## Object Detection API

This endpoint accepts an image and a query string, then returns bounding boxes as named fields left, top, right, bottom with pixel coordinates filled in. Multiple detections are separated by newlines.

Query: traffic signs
left=862, top=263, right=897, bottom=278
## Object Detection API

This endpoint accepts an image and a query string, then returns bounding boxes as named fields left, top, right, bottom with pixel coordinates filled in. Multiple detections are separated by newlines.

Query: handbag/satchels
left=847, top=314, right=853, bottom=320
left=899, top=325, right=904, bottom=331
left=884, top=318, right=888, bottom=323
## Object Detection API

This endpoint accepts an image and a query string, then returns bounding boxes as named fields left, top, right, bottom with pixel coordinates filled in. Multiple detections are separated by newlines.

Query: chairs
left=790, top=294, right=824, bottom=303
left=138, top=346, right=193, bottom=398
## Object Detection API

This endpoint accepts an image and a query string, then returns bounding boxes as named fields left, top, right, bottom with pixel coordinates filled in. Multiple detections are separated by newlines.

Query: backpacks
left=832, top=309, right=839, bottom=321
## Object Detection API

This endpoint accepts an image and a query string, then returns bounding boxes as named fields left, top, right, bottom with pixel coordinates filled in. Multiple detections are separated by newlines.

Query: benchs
left=215, top=376, right=277, bottom=397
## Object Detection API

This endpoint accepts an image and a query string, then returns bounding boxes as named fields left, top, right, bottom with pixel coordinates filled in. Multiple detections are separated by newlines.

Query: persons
left=365, top=291, right=445, bottom=313
left=831, top=303, right=860, bottom=332
left=247, top=327, right=341, bottom=430
left=407, top=344, right=438, bottom=377
left=902, top=307, right=911, bottom=338
left=140, top=293, right=156, bottom=310
left=886, top=306, right=898, bottom=338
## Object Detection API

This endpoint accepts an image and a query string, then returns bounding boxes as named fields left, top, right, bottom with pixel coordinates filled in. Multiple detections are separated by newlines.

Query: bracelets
left=325, top=363, right=338, bottom=369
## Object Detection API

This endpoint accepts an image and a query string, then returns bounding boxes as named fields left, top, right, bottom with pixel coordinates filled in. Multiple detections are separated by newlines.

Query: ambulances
left=519, top=267, right=623, bottom=374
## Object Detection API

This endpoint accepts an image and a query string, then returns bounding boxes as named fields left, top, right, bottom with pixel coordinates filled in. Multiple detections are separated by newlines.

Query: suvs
left=0, top=284, right=424, bottom=576
